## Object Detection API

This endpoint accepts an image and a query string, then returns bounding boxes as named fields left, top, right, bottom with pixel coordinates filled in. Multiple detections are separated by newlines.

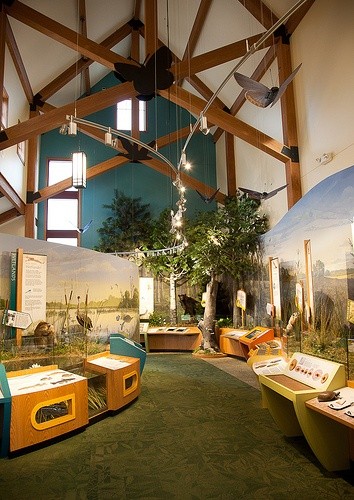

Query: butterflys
left=113, top=46, right=175, bottom=102
left=115, top=136, right=156, bottom=163
left=233, top=62, right=303, bottom=108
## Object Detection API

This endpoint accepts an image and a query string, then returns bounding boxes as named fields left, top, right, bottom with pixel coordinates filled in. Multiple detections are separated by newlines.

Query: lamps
left=72, top=119, right=87, bottom=189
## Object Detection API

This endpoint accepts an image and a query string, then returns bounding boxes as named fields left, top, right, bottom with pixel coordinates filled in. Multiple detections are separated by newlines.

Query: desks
left=110, top=333, right=146, bottom=376
left=0, top=364, right=11, bottom=458
left=141, top=326, right=203, bottom=351
left=259, top=352, right=354, bottom=472
left=5, top=351, right=142, bottom=458
left=219, top=326, right=290, bottom=376
